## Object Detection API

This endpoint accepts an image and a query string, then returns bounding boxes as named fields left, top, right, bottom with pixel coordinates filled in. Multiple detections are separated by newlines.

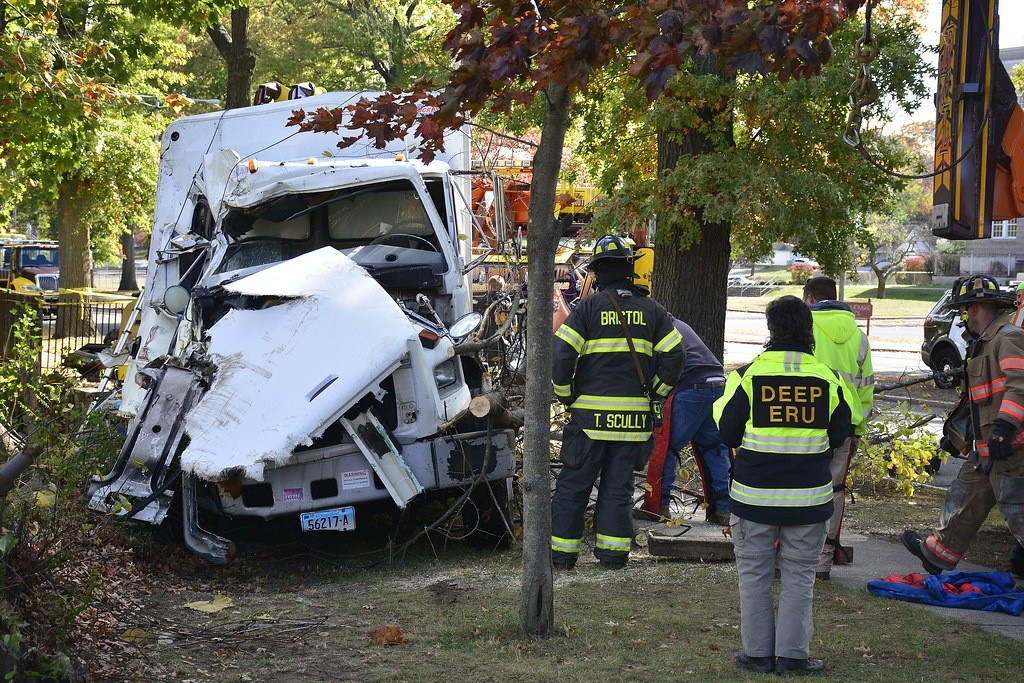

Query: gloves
left=987, top=419, right=1016, bottom=460
left=941, top=438, right=960, bottom=458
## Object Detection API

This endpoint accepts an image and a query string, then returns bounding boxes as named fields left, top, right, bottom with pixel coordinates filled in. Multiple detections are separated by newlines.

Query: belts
left=688, top=382, right=725, bottom=388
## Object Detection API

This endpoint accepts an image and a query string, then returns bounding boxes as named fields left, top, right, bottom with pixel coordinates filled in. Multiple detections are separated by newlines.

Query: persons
left=636, top=313, right=733, bottom=527
left=774, top=277, right=873, bottom=584
left=478, top=269, right=529, bottom=371
left=550, top=233, right=688, bottom=571
left=711, top=295, right=852, bottom=672
left=895, top=273, right=1024, bottom=580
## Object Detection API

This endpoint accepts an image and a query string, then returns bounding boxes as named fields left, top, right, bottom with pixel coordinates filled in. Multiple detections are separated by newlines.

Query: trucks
left=0, top=233, right=59, bottom=316
left=67, top=80, right=529, bottom=569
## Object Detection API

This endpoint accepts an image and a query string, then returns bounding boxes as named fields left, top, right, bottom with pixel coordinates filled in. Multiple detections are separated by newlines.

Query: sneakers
left=735, top=652, right=776, bottom=674
left=776, top=657, right=824, bottom=675
left=901, top=530, right=943, bottom=576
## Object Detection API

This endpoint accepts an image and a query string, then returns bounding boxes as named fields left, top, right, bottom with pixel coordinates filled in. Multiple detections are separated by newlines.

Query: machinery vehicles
left=471, top=159, right=610, bottom=235
left=843, top=0, right=1024, bottom=240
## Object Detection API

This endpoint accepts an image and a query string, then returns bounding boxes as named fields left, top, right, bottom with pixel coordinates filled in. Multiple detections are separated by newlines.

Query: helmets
left=587, top=234, right=646, bottom=272
left=944, top=274, right=1018, bottom=311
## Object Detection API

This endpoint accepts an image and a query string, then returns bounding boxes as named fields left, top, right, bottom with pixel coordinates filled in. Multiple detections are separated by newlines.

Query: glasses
left=805, top=279, right=816, bottom=299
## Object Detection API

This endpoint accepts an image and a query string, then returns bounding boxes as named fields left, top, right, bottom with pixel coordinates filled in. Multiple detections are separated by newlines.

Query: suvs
left=919, top=284, right=1023, bottom=389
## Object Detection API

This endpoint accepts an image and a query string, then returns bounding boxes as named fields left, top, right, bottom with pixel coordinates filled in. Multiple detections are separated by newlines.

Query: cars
left=792, top=257, right=820, bottom=269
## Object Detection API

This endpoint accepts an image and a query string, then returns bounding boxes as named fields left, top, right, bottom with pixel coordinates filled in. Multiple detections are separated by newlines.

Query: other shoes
left=774, top=568, right=782, bottom=578
left=599, top=560, right=624, bottom=570
left=1009, top=559, right=1024, bottom=578
left=553, top=559, right=574, bottom=570
left=816, top=571, right=830, bottom=579
